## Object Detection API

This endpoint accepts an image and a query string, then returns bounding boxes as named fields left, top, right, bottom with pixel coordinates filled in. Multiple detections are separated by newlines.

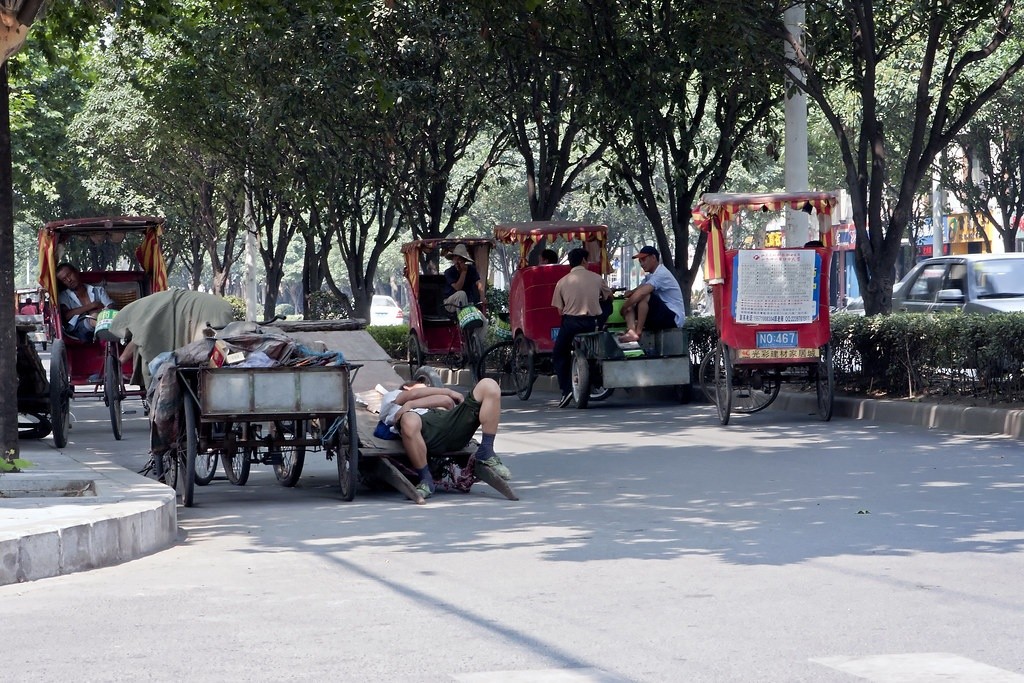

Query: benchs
left=419, top=274, right=458, bottom=324
left=62, top=271, right=149, bottom=345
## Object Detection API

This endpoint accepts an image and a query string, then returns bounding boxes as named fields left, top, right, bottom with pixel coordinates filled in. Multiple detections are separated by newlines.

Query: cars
left=829, top=295, right=865, bottom=319
left=892, top=251, right=1024, bottom=322
left=345, top=295, right=403, bottom=327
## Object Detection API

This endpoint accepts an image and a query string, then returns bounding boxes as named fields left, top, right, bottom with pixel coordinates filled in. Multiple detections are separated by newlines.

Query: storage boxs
left=225, top=351, right=245, bottom=364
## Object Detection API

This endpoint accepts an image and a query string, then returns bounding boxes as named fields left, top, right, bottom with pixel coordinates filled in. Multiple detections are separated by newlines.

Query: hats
left=631, top=245, right=660, bottom=260
left=445, top=243, right=475, bottom=263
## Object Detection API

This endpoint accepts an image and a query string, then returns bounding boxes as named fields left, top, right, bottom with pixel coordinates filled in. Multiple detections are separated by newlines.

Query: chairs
left=948, top=279, right=964, bottom=292
left=927, top=277, right=949, bottom=299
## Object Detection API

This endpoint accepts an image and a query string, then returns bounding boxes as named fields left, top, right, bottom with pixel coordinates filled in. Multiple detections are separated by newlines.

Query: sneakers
left=558, top=390, right=573, bottom=409
left=415, top=483, right=433, bottom=500
left=474, top=456, right=512, bottom=481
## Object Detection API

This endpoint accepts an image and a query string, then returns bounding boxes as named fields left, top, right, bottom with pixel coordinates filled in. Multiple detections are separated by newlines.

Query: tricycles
left=13, top=286, right=50, bottom=350
left=34, top=213, right=170, bottom=442
left=476, top=220, right=614, bottom=401
left=692, top=189, right=845, bottom=426
left=400, top=236, right=497, bottom=382
left=152, top=314, right=366, bottom=510
left=569, top=287, right=697, bottom=410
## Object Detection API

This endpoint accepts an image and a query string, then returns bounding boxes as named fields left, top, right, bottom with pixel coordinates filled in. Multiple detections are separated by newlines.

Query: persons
left=21, top=298, right=38, bottom=315
left=57, top=262, right=134, bottom=364
left=379, top=378, right=511, bottom=500
left=619, top=246, right=685, bottom=342
left=539, top=249, right=558, bottom=265
left=442, top=244, right=487, bottom=353
left=552, top=248, right=613, bottom=408
